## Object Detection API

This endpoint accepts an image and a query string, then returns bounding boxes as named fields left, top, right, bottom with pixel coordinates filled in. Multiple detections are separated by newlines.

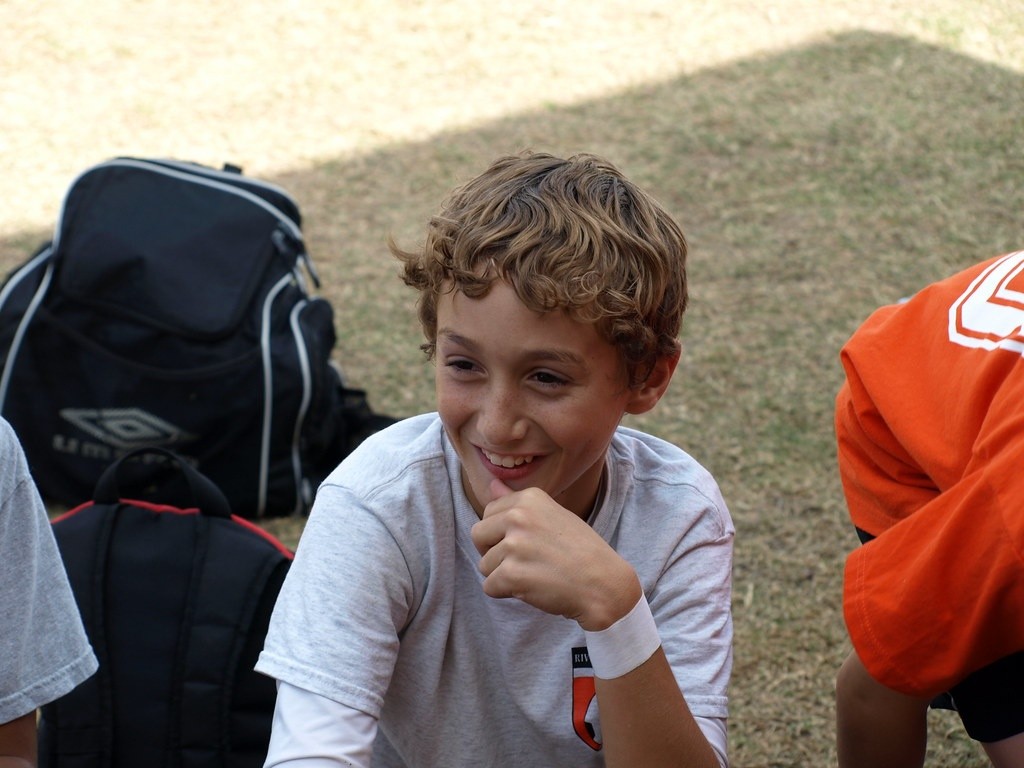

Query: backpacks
left=0, top=154, right=375, bottom=520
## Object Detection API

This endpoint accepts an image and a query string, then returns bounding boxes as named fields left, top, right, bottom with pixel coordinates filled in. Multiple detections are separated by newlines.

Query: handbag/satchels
left=48, top=447, right=295, bottom=767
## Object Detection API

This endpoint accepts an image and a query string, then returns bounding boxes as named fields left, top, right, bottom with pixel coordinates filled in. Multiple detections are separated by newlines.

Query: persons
left=251, top=151, right=734, bottom=768
left=836, top=252, right=1024, bottom=768
left=1, top=412, right=100, bottom=768
